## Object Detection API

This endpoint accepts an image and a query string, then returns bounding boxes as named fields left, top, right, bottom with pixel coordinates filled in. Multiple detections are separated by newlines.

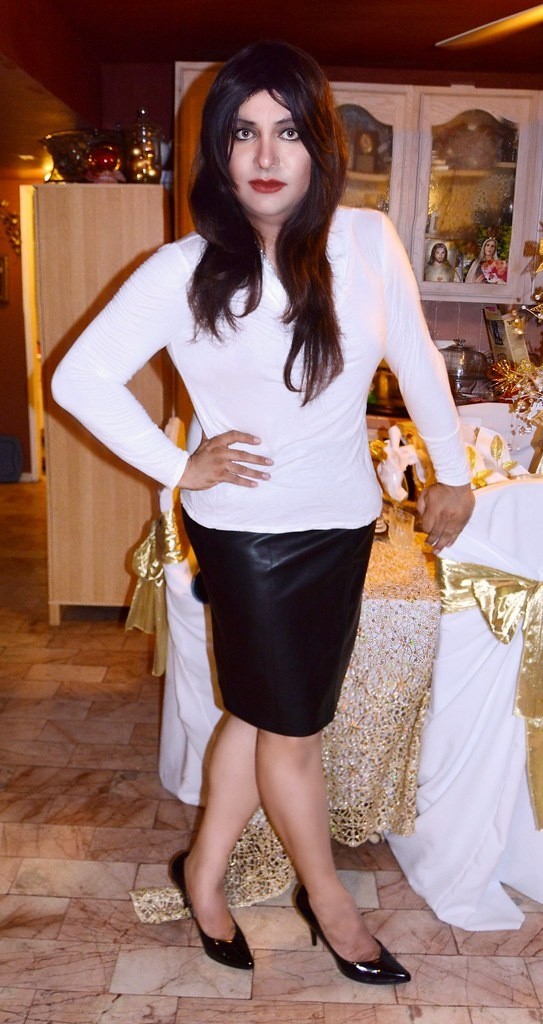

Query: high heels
left=295, top=885, right=412, bottom=985
left=171, top=851, right=255, bottom=969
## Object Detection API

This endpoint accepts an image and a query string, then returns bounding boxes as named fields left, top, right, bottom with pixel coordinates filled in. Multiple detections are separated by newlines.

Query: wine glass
left=93, top=142, right=118, bottom=183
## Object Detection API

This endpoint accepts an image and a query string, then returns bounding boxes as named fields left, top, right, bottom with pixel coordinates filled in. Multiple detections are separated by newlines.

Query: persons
left=50, top=41, right=476, bottom=984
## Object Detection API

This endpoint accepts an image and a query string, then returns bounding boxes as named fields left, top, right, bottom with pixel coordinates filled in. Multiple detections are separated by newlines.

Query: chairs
left=121, top=416, right=444, bottom=924
left=378, top=475, right=542, bottom=931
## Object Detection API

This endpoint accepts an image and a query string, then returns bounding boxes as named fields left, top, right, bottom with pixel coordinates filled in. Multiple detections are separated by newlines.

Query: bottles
left=127, top=106, right=162, bottom=186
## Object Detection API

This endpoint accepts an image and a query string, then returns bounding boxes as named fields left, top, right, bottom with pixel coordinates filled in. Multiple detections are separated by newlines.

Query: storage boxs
left=483, top=305, right=530, bottom=363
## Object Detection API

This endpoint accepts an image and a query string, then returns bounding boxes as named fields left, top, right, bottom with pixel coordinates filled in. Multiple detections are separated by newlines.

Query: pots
left=439, top=338, right=488, bottom=394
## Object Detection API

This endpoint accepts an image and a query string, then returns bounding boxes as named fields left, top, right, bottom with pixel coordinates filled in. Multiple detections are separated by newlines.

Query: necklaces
left=260, top=248, right=285, bottom=289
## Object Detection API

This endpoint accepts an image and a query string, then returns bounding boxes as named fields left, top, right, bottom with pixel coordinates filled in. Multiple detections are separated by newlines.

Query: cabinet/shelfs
left=173, top=60, right=543, bottom=304
left=33, top=182, right=170, bottom=626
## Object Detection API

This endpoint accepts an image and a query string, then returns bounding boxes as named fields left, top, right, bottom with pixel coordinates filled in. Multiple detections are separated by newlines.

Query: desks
left=455, top=403, right=542, bottom=475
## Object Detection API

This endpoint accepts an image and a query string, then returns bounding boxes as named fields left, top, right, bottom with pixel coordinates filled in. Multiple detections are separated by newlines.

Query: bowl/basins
left=36, top=128, right=126, bottom=180
